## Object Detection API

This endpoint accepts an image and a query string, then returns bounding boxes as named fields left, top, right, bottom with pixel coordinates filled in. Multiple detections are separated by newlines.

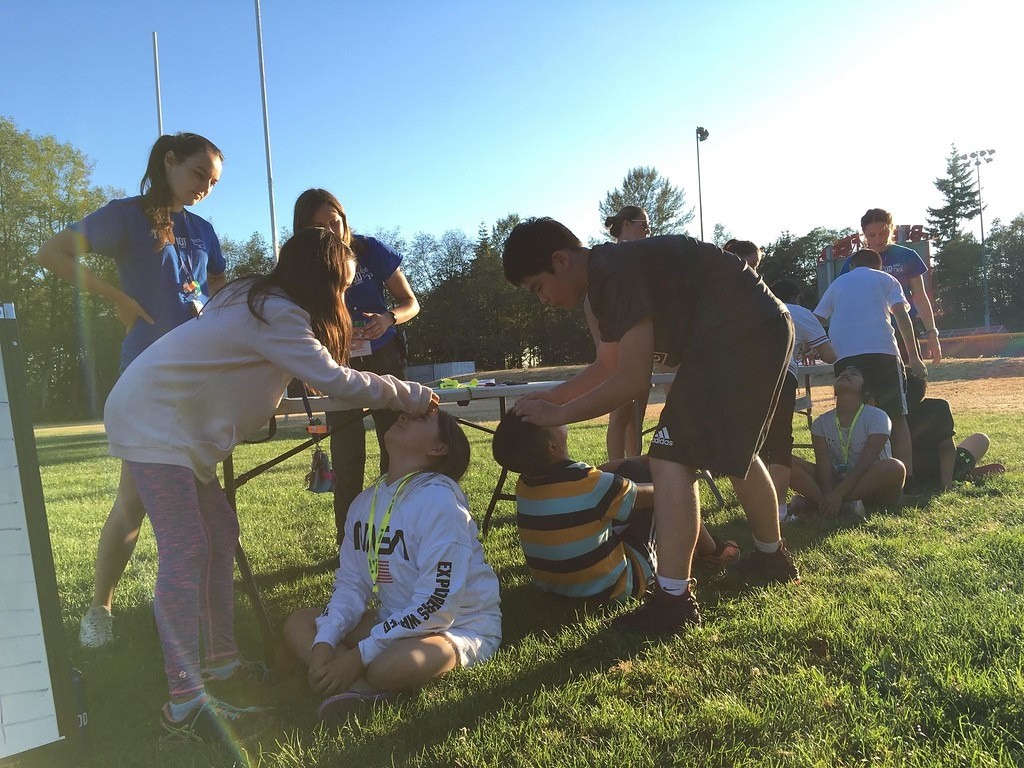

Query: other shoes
left=965, top=464, right=1006, bottom=482
left=904, top=482, right=927, bottom=497
left=317, top=688, right=395, bottom=731
left=789, top=495, right=817, bottom=515
left=841, top=500, right=865, bottom=517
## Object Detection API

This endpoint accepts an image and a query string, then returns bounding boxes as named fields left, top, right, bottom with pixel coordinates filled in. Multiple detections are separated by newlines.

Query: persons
left=105, top=190, right=1005, bottom=744
left=35, top=133, right=222, bottom=651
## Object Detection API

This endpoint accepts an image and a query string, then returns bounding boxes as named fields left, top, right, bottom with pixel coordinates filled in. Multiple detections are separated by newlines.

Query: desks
left=224, top=380, right=565, bottom=669
left=643, top=363, right=836, bottom=507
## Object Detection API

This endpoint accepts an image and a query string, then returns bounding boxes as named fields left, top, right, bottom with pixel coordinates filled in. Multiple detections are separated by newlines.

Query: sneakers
left=202, top=660, right=283, bottom=713
left=158, top=693, right=276, bottom=747
left=779, top=512, right=822, bottom=538
left=611, top=574, right=701, bottom=629
left=692, top=536, right=741, bottom=570
left=78, top=602, right=115, bottom=648
left=724, top=539, right=797, bottom=582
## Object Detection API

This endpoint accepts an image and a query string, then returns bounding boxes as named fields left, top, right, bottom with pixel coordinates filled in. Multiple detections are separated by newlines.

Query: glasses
left=631, top=220, right=650, bottom=234
